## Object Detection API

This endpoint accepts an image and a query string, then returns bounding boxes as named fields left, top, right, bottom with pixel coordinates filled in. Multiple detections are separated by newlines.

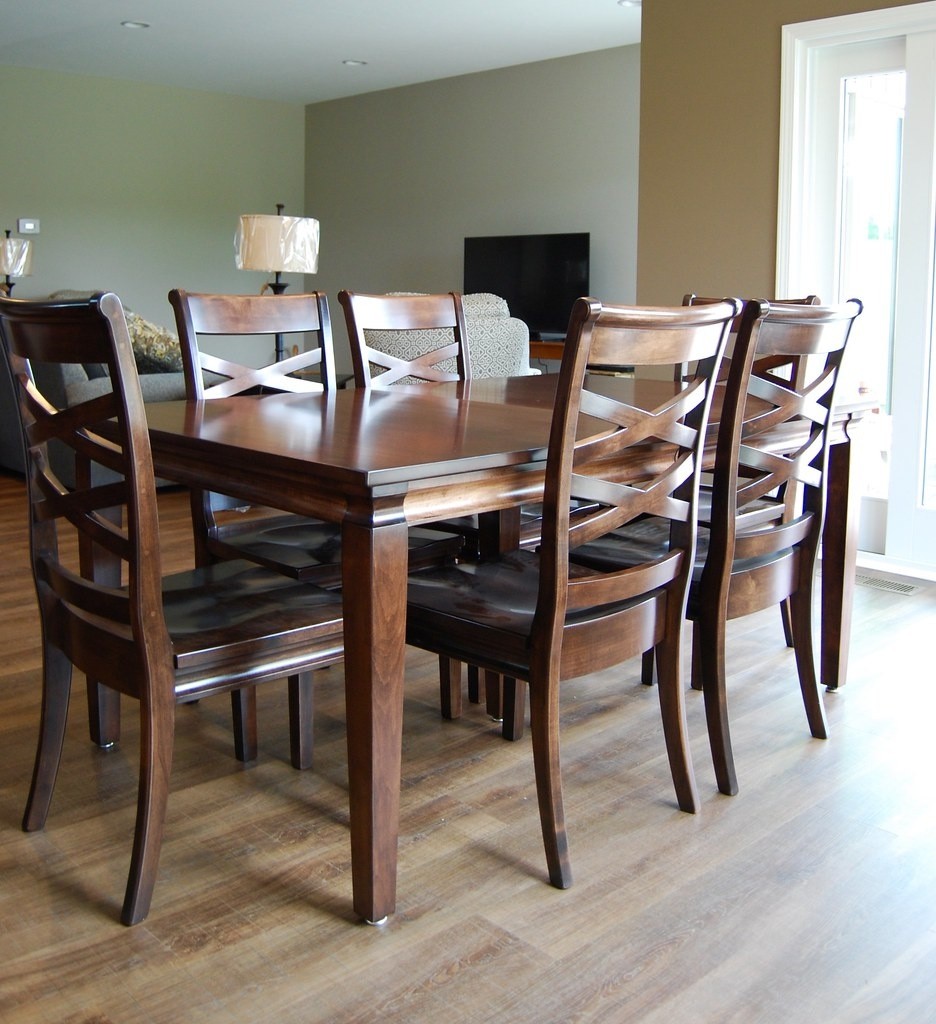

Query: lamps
left=239, top=204, right=320, bottom=362
left=0, top=229, right=34, bottom=297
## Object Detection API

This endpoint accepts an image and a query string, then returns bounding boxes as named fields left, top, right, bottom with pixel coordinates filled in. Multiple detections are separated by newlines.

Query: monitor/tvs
left=463, top=232, right=590, bottom=342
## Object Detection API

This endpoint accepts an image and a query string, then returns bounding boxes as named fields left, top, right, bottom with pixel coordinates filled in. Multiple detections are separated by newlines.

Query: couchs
left=363, top=292, right=541, bottom=385
left=1, top=288, right=232, bottom=492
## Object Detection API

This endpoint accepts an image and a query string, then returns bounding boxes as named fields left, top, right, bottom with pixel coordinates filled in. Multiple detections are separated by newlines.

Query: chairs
left=537, top=297, right=864, bottom=796
left=168, top=288, right=467, bottom=770
left=405, top=296, right=742, bottom=890
left=339, top=289, right=601, bottom=723
left=607, top=293, right=821, bottom=693
left=0, top=293, right=347, bottom=926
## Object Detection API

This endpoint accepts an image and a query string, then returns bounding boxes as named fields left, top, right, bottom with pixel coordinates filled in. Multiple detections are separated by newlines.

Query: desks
left=529, top=342, right=565, bottom=360
left=75, top=374, right=862, bottom=924
